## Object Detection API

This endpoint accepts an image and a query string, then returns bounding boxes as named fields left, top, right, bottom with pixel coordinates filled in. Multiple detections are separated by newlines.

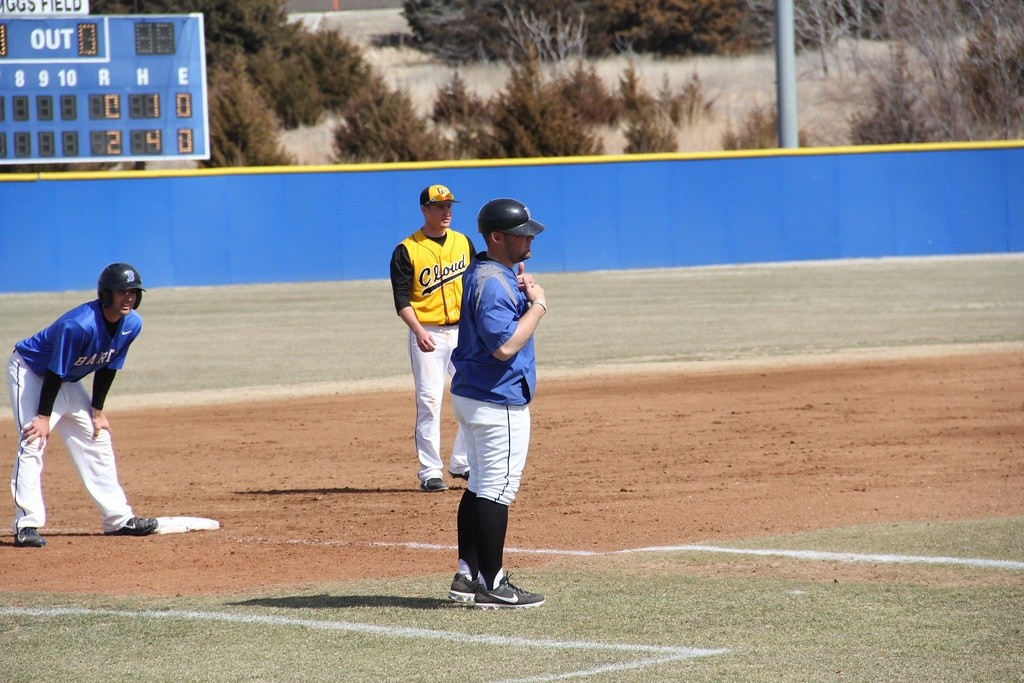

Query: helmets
left=95, top=263, right=148, bottom=311
left=476, top=198, right=545, bottom=236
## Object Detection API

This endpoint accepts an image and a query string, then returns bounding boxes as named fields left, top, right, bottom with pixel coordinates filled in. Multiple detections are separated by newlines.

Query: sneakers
left=447, top=573, right=477, bottom=604
left=472, top=570, right=547, bottom=608
left=419, top=477, right=454, bottom=493
left=12, top=526, right=45, bottom=548
left=101, top=516, right=159, bottom=536
left=447, top=468, right=473, bottom=481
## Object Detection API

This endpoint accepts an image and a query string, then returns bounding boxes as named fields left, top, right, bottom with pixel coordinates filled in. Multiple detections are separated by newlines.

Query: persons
left=8, top=263, right=159, bottom=546
left=448, top=198, right=547, bottom=610
left=389, top=184, right=478, bottom=492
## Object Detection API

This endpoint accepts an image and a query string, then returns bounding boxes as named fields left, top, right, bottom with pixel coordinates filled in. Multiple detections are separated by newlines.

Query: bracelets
left=533, top=300, right=547, bottom=312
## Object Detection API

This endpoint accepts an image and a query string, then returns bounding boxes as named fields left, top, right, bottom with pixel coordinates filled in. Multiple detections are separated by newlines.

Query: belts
left=439, top=320, right=460, bottom=327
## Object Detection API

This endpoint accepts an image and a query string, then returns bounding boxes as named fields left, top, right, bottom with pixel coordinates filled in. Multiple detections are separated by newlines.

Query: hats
left=419, top=185, right=462, bottom=205
